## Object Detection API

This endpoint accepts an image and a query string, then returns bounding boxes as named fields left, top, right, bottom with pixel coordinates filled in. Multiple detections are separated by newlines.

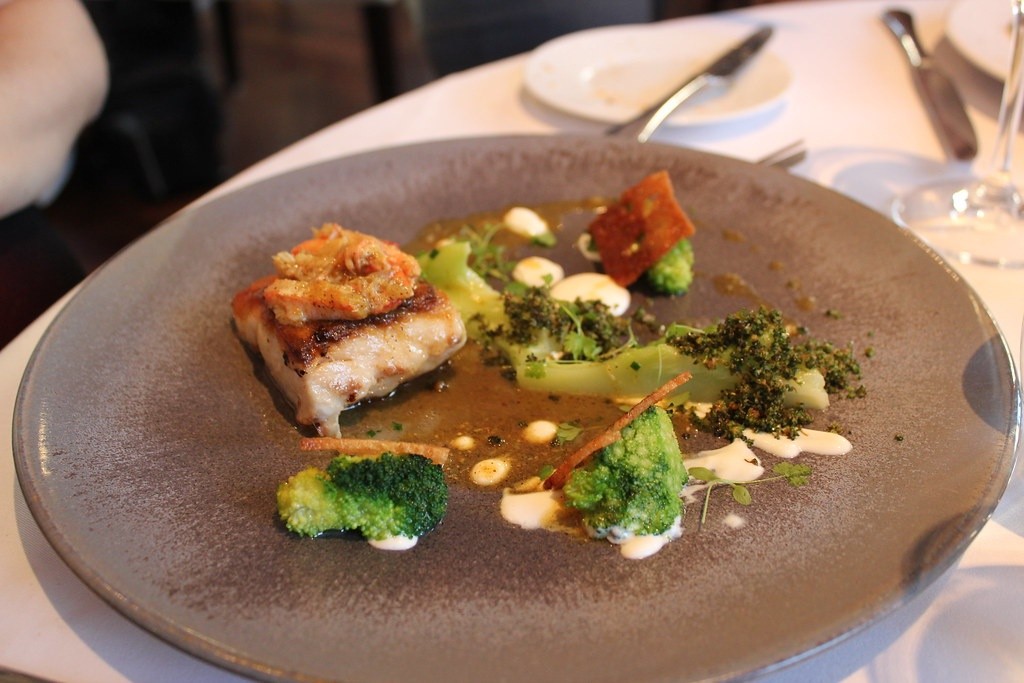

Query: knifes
left=883, top=9, right=983, bottom=161
left=602, top=23, right=776, bottom=146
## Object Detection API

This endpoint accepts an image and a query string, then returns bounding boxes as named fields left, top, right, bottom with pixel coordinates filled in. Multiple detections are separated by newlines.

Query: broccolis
left=564, top=407, right=767, bottom=533
left=413, top=223, right=831, bottom=412
left=273, top=450, right=447, bottom=538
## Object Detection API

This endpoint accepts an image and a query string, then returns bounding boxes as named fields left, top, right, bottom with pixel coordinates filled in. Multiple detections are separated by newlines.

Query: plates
left=12, top=133, right=1023, bottom=683
left=524, top=24, right=796, bottom=131
left=946, top=0, right=1024, bottom=99
